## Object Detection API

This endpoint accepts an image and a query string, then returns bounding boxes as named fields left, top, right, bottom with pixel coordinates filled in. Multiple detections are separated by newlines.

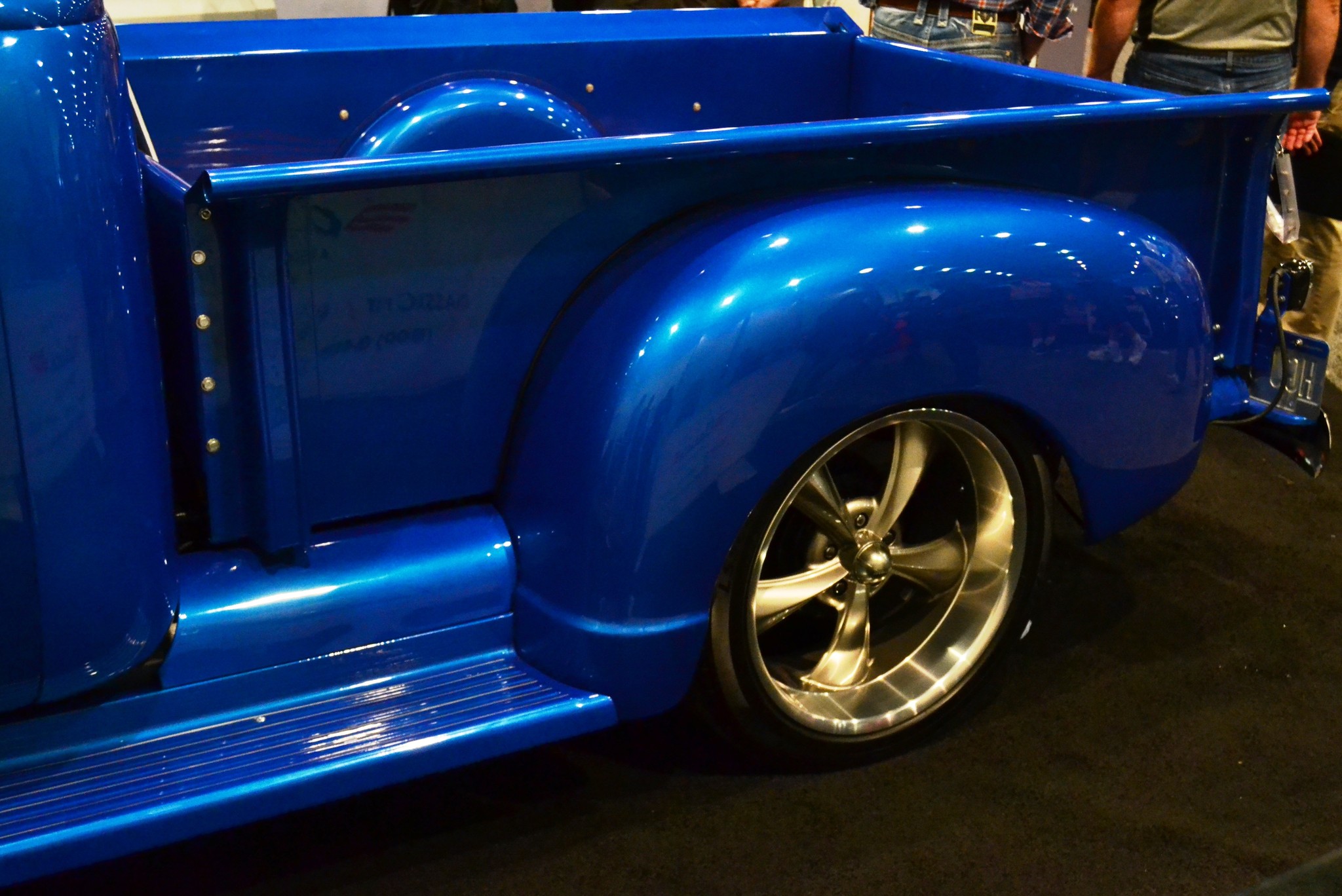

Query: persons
left=386, top=0, right=873, bottom=36
left=858, top=0, right=1076, bottom=68
left=1082, top=1, right=1340, bottom=148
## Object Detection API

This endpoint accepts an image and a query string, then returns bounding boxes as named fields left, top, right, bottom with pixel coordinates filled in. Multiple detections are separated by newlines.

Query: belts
left=1149, top=38, right=1285, bottom=54
left=876, top=0, right=1019, bottom=24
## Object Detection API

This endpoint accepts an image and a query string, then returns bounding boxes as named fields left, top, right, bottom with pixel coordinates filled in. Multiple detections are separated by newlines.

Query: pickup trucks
left=0, top=0, right=1329, bottom=892
